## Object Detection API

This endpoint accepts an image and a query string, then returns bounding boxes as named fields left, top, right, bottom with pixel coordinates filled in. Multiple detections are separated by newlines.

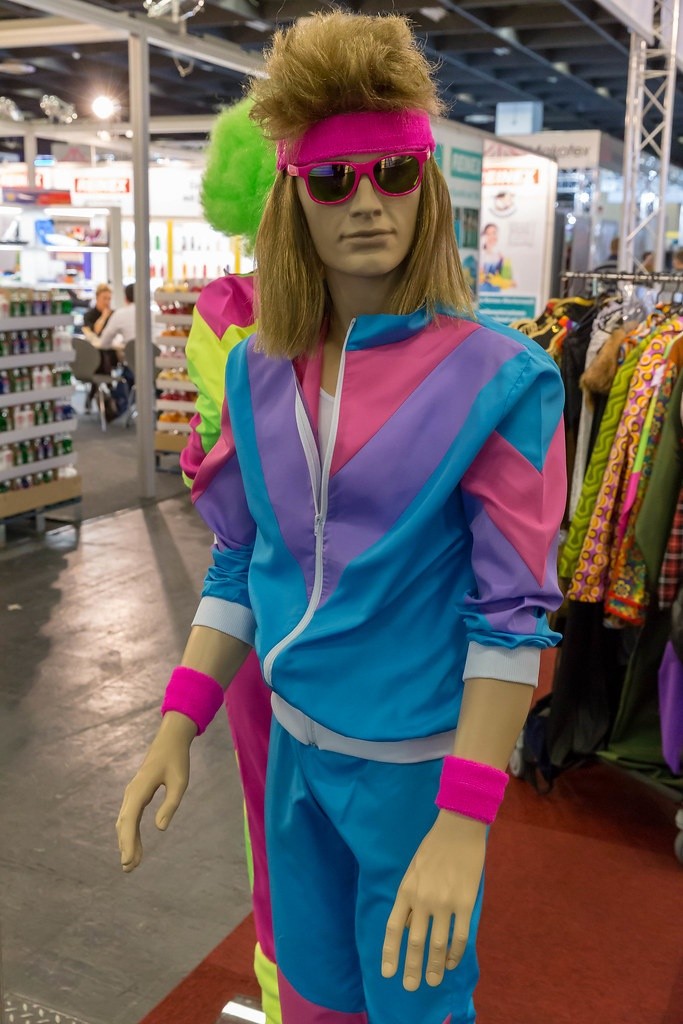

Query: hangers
left=561, top=270, right=683, bottom=337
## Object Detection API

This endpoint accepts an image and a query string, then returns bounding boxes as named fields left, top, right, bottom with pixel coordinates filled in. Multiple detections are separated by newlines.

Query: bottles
left=0, top=432, right=77, bottom=493
left=0, top=290, right=74, bottom=318
left=0, top=397, right=76, bottom=432
left=0, top=364, right=72, bottom=394
left=0, top=329, right=52, bottom=357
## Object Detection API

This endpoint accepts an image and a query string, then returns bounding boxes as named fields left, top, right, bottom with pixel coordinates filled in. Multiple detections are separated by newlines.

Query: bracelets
left=162, top=666, right=223, bottom=735
left=435, top=756, right=509, bottom=825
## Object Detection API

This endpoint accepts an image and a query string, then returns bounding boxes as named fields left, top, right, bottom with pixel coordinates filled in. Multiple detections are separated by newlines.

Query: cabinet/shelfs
left=0, top=282, right=81, bottom=524
left=154, top=280, right=214, bottom=465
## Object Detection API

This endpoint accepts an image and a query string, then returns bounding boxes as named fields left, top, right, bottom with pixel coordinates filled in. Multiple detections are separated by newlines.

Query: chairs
left=123, top=340, right=160, bottom=429
left=71, top=336, right=124, bottom=432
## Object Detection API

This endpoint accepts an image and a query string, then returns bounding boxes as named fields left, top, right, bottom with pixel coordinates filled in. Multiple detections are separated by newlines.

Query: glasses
left=288, top=147, right=431, bottom=204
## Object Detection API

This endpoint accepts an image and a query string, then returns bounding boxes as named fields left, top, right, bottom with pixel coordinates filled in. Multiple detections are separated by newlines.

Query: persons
left=478, top=223, right=503, bottom=292
left=596, top=237, right=683, bottom=278
left=115, top=7, right=577, bottom=1024
left=83, top=283, right=136, bottom=427
